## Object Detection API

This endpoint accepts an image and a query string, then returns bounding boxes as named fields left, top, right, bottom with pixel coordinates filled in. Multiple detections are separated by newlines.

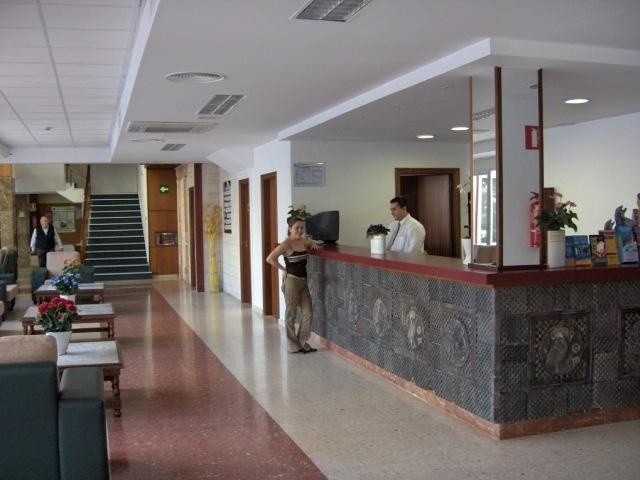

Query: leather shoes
left=304, top=348, right=317, bottom=353
left=294, top=349, right=305, bottom=353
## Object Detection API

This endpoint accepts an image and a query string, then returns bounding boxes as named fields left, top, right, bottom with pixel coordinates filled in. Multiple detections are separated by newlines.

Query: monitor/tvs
left=306, top=210, right=339, bottom=244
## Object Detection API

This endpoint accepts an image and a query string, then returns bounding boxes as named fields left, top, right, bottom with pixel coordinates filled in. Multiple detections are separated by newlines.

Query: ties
left=388, top=222, right=401, bottom=251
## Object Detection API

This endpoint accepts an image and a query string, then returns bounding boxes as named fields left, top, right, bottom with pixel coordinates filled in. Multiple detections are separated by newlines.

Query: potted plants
left=544, top=198, right=578, bottom=268
left=367, top=224, right=389, bottom=256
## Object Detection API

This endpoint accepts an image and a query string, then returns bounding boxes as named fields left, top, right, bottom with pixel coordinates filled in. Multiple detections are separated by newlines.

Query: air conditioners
left=124, top=121, right=219, bottom=135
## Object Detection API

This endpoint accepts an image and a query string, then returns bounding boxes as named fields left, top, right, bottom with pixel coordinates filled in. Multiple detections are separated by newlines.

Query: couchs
left=29, top=252, right=95, bottom=305
left=0, top=245, right=20, bottom=326
left=2, top=335, right=109, bottom=480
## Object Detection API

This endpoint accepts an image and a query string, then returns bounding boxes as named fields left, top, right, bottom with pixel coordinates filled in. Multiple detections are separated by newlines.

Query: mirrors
left=471, top=70, right=497, bottom=267
left=501, top=68, right=543, bottom=267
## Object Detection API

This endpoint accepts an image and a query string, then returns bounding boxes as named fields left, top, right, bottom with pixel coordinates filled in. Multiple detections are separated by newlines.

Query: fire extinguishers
left=528, top=191, right=541, bottom=248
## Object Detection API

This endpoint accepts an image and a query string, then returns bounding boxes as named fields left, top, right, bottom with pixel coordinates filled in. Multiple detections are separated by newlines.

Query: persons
left=266, top=217, right=322, bottom=354
left=30, top=216, right=63, bottom=268
left=384, top=196, right=426, bottom=255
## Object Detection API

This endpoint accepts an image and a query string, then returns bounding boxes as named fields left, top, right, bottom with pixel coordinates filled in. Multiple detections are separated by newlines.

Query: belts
left=287, top=273, right=305, bottom=280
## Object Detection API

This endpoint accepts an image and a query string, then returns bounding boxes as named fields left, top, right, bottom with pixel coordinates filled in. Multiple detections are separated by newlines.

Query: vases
left=44, top=331, right=73, bottom=356
left=58, top=294, right=76, bottom=305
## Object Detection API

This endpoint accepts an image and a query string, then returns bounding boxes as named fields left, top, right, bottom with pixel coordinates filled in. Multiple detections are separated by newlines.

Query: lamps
left=198, top=95, right=245, bottom=116
left=296, top=0, right=373, bottom=23
left=161, top=143, right=187, bottom=152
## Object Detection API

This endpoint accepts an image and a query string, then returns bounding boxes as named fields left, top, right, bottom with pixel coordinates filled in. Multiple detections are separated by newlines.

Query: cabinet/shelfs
left=296, top=241, right=640, bottom=440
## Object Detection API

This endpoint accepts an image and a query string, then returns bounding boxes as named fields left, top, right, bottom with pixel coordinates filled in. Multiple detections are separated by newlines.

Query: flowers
left=52, top=272, right=83, bottom=294
left=34, top=298, right=83, bottom=332
left=62, top=255, right=82, bottom=274
